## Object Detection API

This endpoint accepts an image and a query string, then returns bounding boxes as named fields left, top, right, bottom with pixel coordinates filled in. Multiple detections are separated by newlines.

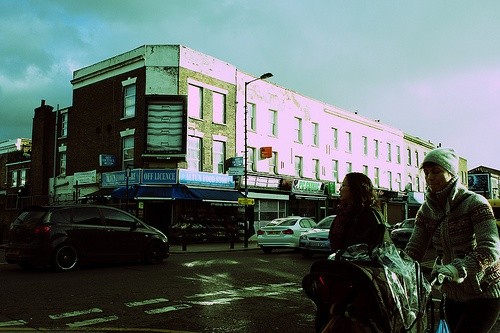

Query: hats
left=423, top=146, right=458, bottom=177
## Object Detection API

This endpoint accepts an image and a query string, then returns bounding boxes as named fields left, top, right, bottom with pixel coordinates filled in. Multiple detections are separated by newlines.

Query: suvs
left=6, top=203, right=170, bottom=275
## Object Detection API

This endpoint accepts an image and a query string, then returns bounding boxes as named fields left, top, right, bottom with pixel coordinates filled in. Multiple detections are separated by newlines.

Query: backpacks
left=331, top=241, right=433, bottom=333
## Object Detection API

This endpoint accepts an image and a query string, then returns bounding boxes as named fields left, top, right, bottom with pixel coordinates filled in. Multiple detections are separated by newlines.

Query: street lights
left=243, top=72, right=275, bottom=249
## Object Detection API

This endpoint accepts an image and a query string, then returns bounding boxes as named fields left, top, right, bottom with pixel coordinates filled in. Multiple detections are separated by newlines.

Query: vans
left=488, top=198, right=500, bottom=236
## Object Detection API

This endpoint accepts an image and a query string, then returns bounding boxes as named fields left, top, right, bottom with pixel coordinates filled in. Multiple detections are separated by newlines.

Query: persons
left=403, top=146, right=500, bottom=333
left=327, top=173, right=393, bottom=254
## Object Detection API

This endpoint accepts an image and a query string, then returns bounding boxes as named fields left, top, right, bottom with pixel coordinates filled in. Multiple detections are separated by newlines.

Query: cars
left=299, top=215, right=337, bottom=256
left=383, top=218, right=417, bottom=249
left=257, top=216, right=316, bottom=254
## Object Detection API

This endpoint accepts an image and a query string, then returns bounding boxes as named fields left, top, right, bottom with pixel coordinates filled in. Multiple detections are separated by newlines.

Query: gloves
left=430, top=262, right=459, bottom=283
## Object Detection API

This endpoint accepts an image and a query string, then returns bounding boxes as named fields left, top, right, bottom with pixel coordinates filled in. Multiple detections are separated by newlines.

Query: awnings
left=78, top=184, right=246, bottom=204
